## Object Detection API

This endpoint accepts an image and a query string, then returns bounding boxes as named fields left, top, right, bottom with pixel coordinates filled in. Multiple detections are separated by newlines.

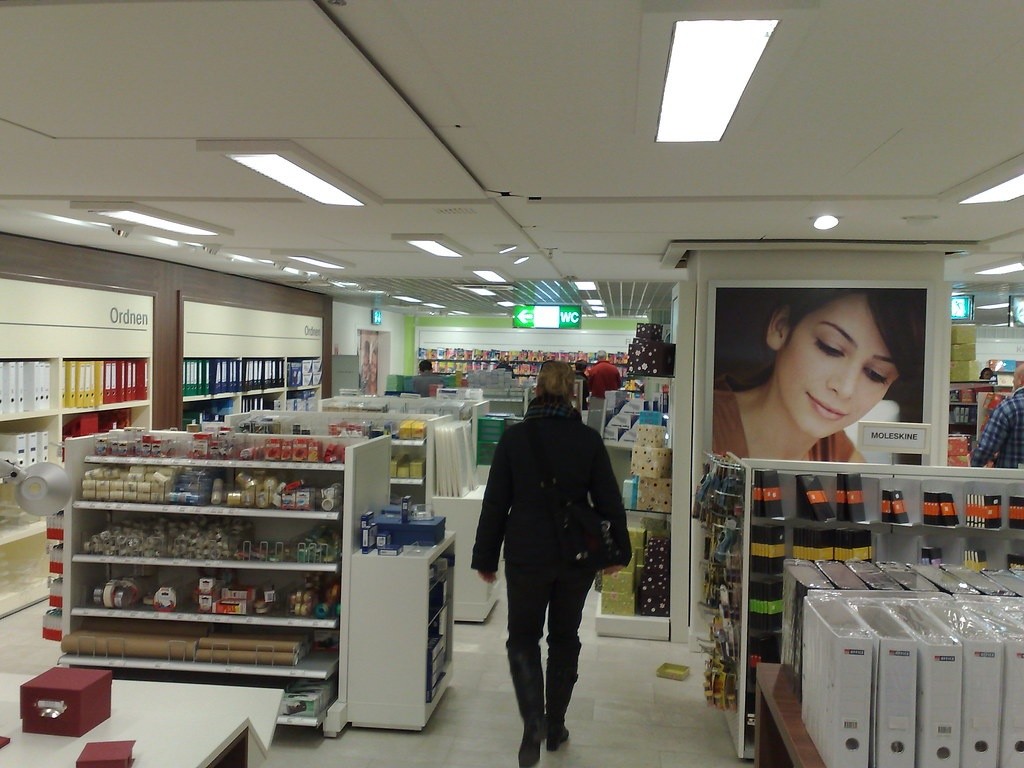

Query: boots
left=544, top=655, right=578, bottom=751
left=506, top=645, right=550, bottom=766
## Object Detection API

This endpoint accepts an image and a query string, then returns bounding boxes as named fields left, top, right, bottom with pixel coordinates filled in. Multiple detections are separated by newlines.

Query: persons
left=413, top=360, right=445, bottom=397
left=979, top=368, right=993, bottom=379
left=587, top=350, right=621, bottom=397
left=572, top=360, right=590, bottom=410
left=970, top=363, right=1024, bottom=469
left=496, top=361, right=515, bottom=379
left=471, top=361, right=631, bottom=768
left=714, top=289, right=926, bottom=467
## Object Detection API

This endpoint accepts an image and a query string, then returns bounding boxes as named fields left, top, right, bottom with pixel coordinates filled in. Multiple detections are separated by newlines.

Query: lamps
left=271, top=248, right=356, bottom=270
left=938, top=152, right=1024, bottom=206
left=494, top=244, right=517, bottom=254
left=512, top=256, right=529, bottom=264
left=391, top=233, right=474, bottom=259
left=464, top=265, right=513, bottom=285
left=196, top=139, right=384, bottom=209
left=0, top=456, right=71, bottom=516
left=68, top=200, right=235, bottom=241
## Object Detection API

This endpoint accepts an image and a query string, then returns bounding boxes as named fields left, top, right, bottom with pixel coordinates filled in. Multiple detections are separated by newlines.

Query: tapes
left=82, top=465, right=211, bottom=506
left=102, top=578, right=119, bottom=608
left=143, top=596, right=155, bottom=605
left=195, top=574, right=341, bottom=617
left=93, top=583, right=104, bottom=605
left=211, top=470, right=278, bottom=506
left=154, top=588, right=178, bottom=612
left=320, top=481, right=343, bottom=512
left=116, top=578, right=142, bottom=603
left=82, top=514, right=340, bottom=564
left=112, top=588, right=133, bottom=607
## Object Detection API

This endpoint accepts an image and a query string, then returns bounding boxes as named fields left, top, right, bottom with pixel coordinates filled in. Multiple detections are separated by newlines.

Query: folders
left=0, top=356, right=285, bottom=414
left=0, top=396, right=266, bottom=471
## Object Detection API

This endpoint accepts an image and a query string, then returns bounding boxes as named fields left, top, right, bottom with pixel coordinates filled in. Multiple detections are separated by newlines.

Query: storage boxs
left=400, top=420, right=414, bottom=440
left=264, top=437, right=283, bottom=460
left=198, top=593, right=220, bottom=614
left=220, top=586, right=261, bottom=602
left=283, top=692, right=320, bottom=717
left=141, top=435, right=155, bottom=457
left=412, top=420, right=427, bottom=439
left=615, top=398, right=649, bottom=413
left=600, top=517, right=671, bottom=616
left=208, top=439, right=229, bottom=460
left=986, top=359, right=1017, bottom=373
left=948, top=437, right=968, bottom=456
left=604, top=426, right=629, bottom=442
left=646, top=307, right=672, bottom=324
left=607, top=413, right=640, bottom=428
left=378, top=545, right=403, bottom=556
left=410, top=457, right=426, bottom=479
left=213, top=598, right=253, bottom=614
left=281, top=439, right=292, bottom=460
left=950, top=323, right=981, bottom=381
left=192, top=432, right=213, bottom=459
left=626, top=322, right=676, bottom=376
left=313, top=629, right=339, bottom=653
left=152, top=439, right=169, bottom=457
left=636, top=476, right=672, bottom=514
left=635, top=424, right=668, bottom=448
left=362, top=523, right=376, bottom=554
left=198, top=577, right=216, bottom=595
left=376, top=532, right=391, bottom=547
left=20, top=667, right=113, bottom=738
left=76, top=740, right=136, bottom=768
left=290, top=685, right=330, bottom=710
left=391, top=453, right=408, bottom=477
left=648, top=400, right=659, bottom=411
left=361, top=511, right=374, bottom=527
left=385, top=369, right=519, bottom=394
left=630, top=447, right=672, bottom=479
left=947, top=451, right=974, bottom=467
left=293, top=438, right=308, bottom=461
left=397, top=461, right=410, bottom=478
left=307, top=440, right=323, bottom=462
left=370, top=513, right=446, bottom=546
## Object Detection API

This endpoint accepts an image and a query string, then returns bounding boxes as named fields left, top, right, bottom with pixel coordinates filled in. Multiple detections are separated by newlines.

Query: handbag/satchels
left=564, top=507, right=623, bottom=572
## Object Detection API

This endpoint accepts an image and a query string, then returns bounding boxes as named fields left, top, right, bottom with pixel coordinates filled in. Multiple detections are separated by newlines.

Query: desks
left=753, top=662, right=827, bottom=768
left=0, top=673, right=283, bottom=768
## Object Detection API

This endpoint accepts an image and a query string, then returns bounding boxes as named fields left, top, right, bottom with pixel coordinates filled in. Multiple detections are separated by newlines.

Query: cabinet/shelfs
left=0, top=356, right=154, bottom=615
left=699, top=450, right=1024, bottom=757
left=419, top=358, right=626, bottom=379
left=948, top=383, right=995, bottom=436
left=183, top=357, right=322, bottom=413
left=64, top=389, right=528, bottom=739
